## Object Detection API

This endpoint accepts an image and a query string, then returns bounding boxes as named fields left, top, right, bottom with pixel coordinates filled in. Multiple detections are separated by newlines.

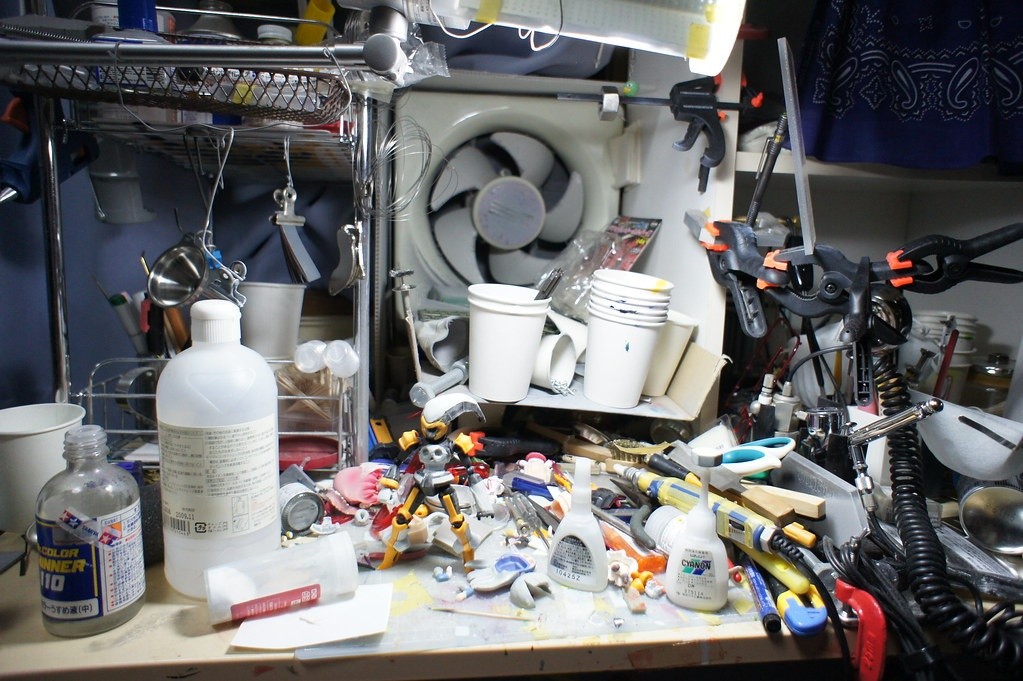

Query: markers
left=112, top=278, right=189, bottom=361
left=744, top=560, right=781, bottom=632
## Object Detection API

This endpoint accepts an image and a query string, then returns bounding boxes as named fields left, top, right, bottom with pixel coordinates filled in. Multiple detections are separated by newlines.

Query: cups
left=0, top=404, right=87, bottom=532
left=240, top=282, right=306, bottom=360
left=642, top=310, right=698, bottom=397
left=915, top=311, right=978, bottom=404
left=584, top=269, right=674, bottom=408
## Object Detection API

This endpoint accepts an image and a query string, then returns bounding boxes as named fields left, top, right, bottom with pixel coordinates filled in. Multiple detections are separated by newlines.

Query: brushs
left=603, top=439, right=825, bottom=519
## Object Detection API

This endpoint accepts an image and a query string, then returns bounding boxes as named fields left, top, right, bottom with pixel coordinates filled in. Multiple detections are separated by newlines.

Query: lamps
left=431, top=0, right=746, bottom=75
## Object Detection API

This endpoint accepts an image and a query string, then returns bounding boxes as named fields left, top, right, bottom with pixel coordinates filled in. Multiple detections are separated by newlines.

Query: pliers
left=756, top=239, right=933, bottom=321
left=885, top=220, right=1023, bottom=296
left=610, top=476, right=658, bottom=549
left=685, top=209, right=784, bottom=338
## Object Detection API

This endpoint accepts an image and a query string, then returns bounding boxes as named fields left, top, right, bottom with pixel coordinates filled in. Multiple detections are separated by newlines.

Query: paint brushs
left=137, top=250, right=150, bottom=275
left=85, top=270, right=109, bottom=302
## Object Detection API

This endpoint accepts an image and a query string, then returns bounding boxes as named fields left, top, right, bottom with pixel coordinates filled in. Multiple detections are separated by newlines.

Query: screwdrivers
left=501, top=487, right=550, bottom=551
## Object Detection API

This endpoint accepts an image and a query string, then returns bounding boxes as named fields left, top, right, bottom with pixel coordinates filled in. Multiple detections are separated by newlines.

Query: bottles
left=156, top=300, right=279, bottom=600
left=36, top=426, right=146, bottom=638
left=467, top=284, right=576, bottom=402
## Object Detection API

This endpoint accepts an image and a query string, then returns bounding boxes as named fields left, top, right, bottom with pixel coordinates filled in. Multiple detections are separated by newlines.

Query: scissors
left=719, top=436, right=796, bottom=478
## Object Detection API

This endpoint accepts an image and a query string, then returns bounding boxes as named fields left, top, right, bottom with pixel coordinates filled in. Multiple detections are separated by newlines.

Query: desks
left=0, top=529, right=1023, bottom=681
